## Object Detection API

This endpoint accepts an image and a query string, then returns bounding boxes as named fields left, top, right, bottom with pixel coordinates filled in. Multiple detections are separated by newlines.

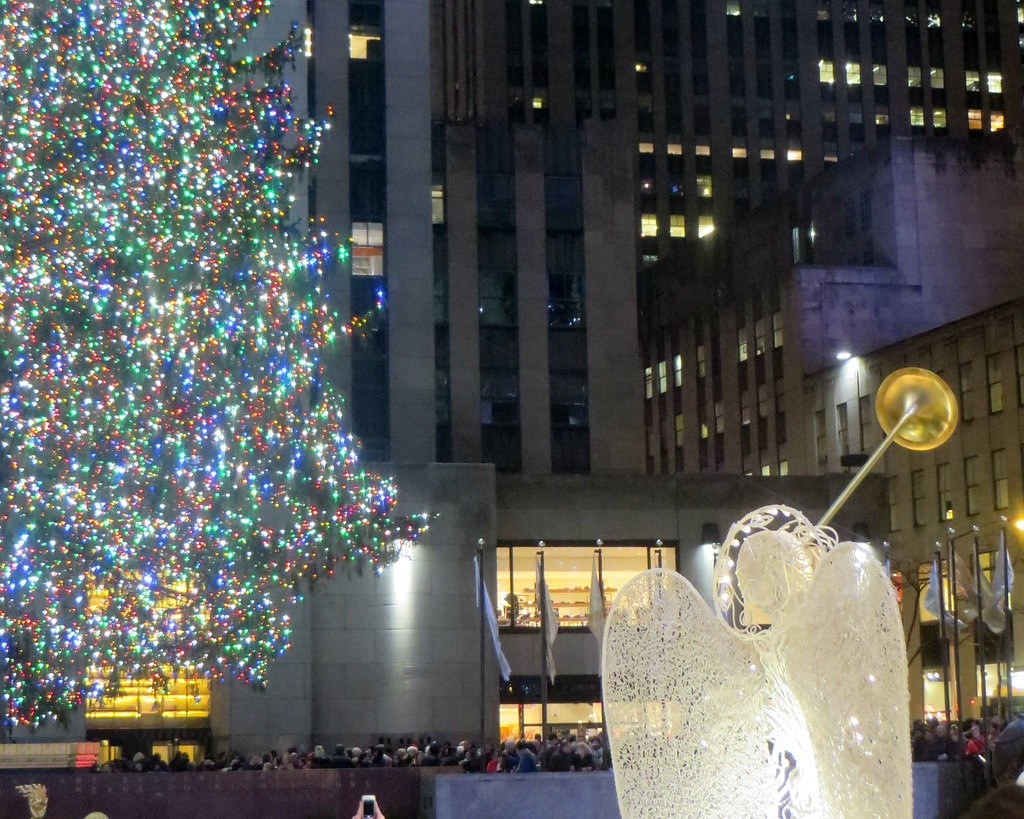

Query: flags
left=589, top=553, right=607, bottom=679
left=534, top=555, right=562, bottom=684
left=925, top=529, right=1015, bottom=640
left=475, top=557, right=514, bottom=683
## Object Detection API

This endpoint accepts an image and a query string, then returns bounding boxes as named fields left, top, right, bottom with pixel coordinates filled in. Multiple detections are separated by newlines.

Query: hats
left=516, top=740, right=528, bottom=750
left=314, top=745, right=324, bottom=758
left=394, top=748, right=408, bottom=760
left=964, top=731, right=974, bottom=740
left=352, top=747, right=362, bottom=756
left=992, top=714, right=1024, bottom=744
left=407, top=746, right=419, bottom=757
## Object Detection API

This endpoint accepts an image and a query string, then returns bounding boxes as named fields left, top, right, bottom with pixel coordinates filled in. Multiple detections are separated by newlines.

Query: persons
left=460, top=750, right=477, bottom=774
left=472, top=732, right=613, bottom=773
left=505, top=742, right=539, bottom=773
left=98, top=736, right=472, bottom=772
left=549, top=740, right=573, bottom=771
left=574, top=743, right=595, bottom=771
left=911, top=711, right=1024, bottom=760
